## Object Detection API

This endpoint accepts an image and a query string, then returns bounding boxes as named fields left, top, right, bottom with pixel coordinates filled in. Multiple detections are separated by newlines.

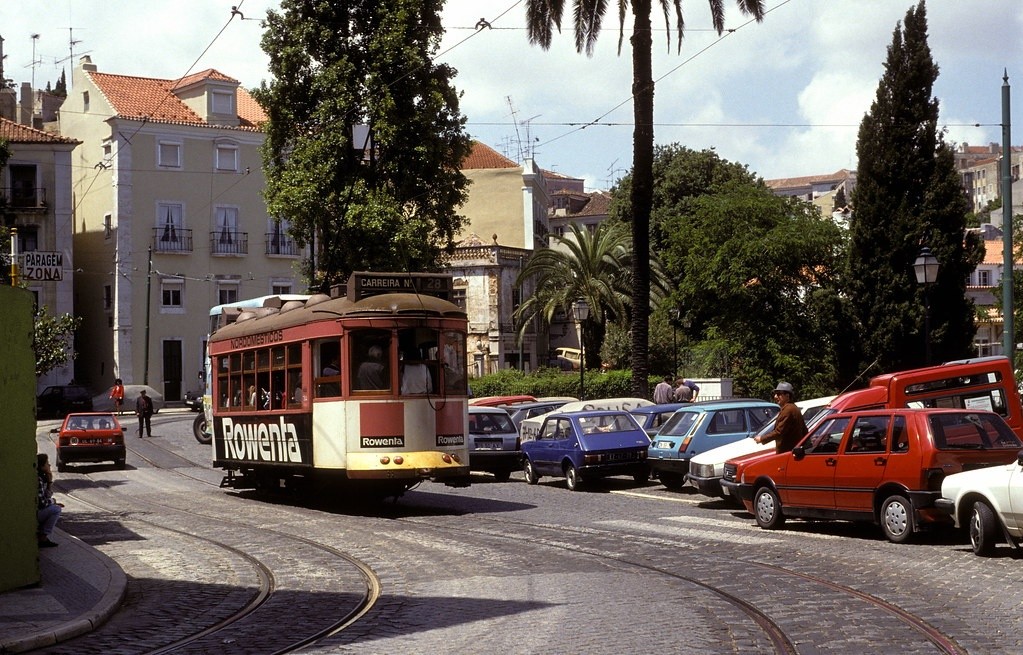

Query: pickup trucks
left=719, top=354, right=1023, bottom=519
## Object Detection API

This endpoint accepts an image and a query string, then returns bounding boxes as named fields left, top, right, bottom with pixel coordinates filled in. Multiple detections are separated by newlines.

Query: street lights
left=912, top=246, right=941, bottom=369
left=573, top=295, right=590, bottom=400
left=669, top=306, right=680, bottom=380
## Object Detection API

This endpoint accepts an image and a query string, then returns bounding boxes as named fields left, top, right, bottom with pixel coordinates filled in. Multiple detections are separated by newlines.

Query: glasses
left=775, top=391, right=786, bottom=396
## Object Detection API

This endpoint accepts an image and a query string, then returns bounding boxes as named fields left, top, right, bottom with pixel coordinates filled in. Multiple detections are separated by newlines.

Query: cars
left=183, top=390, right=205, bottom=411
left=468, top=395, right=655, bottom=491
left=736, top=408, right=1023, bottom=542
left=688, top=394, right=926, bottom=507
left=551, top=357, right=573, bottom=371
left=51, top=412, right=127, bottom=472
left=92, top=385, right=163, bottom=415
left=646, top=397, right=783, bottom=492
left=934, top=448, right=1023, bottom=556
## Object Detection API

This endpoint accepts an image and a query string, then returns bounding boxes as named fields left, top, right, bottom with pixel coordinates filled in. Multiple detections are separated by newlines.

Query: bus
left=204, top=295, right=471, bottom=504
left=193, top=294, right=329, bottom=444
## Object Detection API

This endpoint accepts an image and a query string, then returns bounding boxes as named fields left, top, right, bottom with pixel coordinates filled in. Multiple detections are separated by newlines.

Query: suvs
left=36, top=386, right=92, bottom=418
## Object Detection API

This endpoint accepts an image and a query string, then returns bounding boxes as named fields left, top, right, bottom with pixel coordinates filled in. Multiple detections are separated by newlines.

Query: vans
left=556, top=347, right=585, bottom=368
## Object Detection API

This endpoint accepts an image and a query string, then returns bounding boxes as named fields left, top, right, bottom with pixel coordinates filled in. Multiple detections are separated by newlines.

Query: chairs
left=853, top=424, right=887, bottom=451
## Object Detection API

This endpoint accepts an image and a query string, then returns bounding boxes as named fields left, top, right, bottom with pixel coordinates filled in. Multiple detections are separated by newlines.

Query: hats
left=772, top=382, right=795, bottom=398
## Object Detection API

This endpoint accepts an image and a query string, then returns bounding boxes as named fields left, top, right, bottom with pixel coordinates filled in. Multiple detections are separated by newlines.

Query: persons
left=755, top=380, right=813, bottom=455
left=654, top=375, right=699, bottom=403
left=36, top=454, right=64, bottom=547
left=110, top=379, right=125, bottom=416
left=135, top=391, right=153, bottom=437
left=220, top=343, right=466, bottom=409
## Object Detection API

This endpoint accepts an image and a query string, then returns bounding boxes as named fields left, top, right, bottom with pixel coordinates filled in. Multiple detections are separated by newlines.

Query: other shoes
left=148, top=434, right=151, bottom=437
left=38, top=539, right=58, bottom=548
left=139, top=434, right=142, bottom=438
